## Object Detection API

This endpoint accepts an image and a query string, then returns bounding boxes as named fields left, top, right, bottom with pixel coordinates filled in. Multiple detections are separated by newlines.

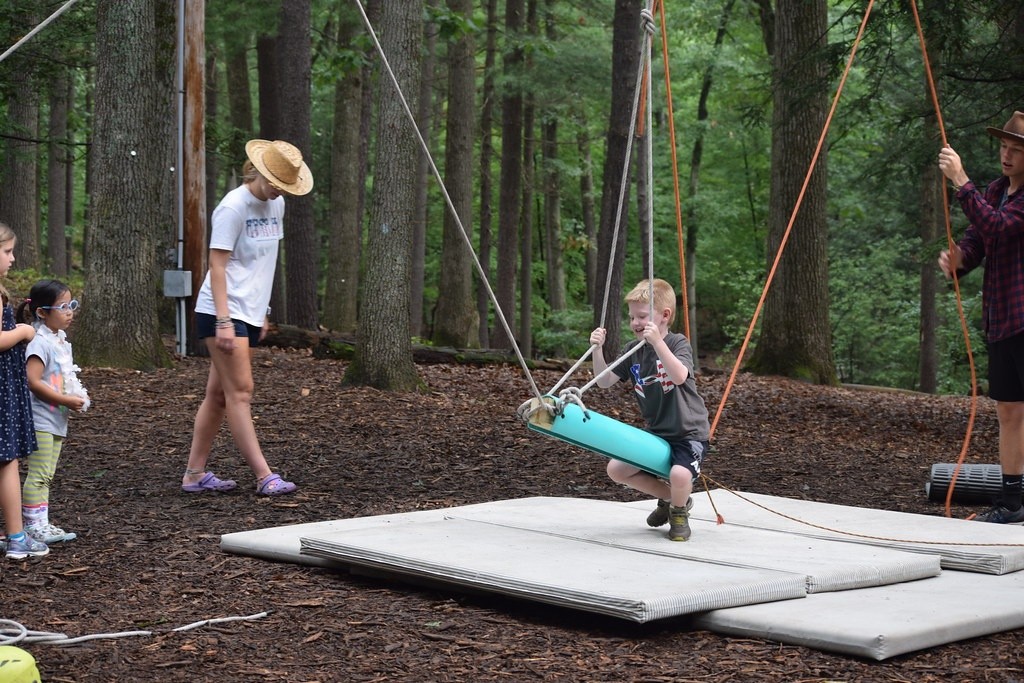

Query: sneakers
left=0, top=541, right=7, bottom=554
left=972, top=504, right=1024, bottom=524
left=646, top=496, right=694, bottom=527
left=24, top=523, right=61, bottom=543
left=667, top=504, right=692, bottom=541
left=6, top=532, right=50, bottom=559
left=43, top=524, right=76, bottom=540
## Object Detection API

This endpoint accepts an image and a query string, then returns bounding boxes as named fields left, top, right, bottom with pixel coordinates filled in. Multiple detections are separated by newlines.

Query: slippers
left=181, top=471, right=236, bottom=492
left=255, top=474, right=297, bottom=495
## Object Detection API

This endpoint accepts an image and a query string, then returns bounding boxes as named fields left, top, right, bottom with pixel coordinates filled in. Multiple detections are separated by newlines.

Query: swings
left=357, top=0, right=874, bottom=488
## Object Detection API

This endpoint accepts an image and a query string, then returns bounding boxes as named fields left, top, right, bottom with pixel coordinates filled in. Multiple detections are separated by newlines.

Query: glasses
left=35, top=300, right=78, bottom=321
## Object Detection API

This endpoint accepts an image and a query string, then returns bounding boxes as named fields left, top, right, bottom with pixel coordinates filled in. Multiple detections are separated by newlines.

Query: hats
left=246, top=139, right=314, bottom=195
left=986, top=111, right=1024, bottom=143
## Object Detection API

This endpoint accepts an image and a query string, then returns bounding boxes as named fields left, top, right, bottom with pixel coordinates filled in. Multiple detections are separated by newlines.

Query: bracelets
left=214, top=316, right=233, bottom=329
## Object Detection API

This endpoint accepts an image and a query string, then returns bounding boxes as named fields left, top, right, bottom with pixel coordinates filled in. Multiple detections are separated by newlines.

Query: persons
left=182, top=139, right=314, bottom=496
left=0, top=223, right=50, bottom=559
left=16, top=279, right=90, bottom=544
left=590, top=279, right=711, bottom=541
left=938, top=111, right=1024, bottom=524
